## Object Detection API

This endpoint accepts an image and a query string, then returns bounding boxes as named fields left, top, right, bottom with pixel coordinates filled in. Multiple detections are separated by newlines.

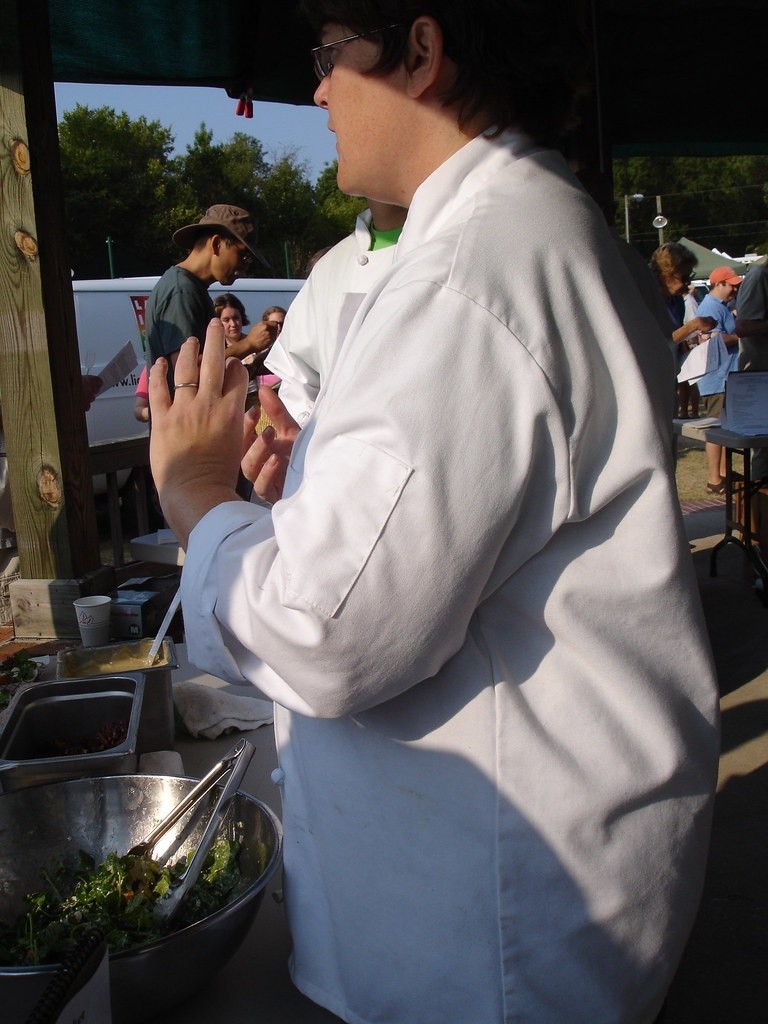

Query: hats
left=688, top=285, right=698, bottom=292
left=708, top=266, right=743, bottom=286
left=172, top=204, right=272, bottom=273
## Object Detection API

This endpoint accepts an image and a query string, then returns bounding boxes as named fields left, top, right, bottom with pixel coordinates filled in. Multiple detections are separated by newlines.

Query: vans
left=1, top=274, right=308, bottom=555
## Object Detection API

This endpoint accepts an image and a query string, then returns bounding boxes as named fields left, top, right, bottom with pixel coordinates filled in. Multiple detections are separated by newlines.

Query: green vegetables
left=0, top=834, right=241, bottom=969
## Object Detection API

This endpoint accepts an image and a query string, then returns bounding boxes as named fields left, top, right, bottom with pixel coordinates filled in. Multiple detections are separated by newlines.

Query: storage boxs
left=106, top=591, right=161, bottom=637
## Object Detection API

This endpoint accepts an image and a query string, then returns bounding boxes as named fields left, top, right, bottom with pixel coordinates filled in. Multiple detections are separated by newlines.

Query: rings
left=173, top=382, right=198, bottom=389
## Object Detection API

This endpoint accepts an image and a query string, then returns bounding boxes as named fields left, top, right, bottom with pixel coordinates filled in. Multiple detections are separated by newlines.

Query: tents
left=676, top=237, right=747, bottom=281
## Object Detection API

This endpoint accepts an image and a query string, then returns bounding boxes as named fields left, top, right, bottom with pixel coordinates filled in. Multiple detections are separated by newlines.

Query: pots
left=0, top=771, right=284, bottom=1023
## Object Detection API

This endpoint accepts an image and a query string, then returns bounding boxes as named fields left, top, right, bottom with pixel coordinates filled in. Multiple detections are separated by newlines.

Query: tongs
left=117, top=738, right=257, bottom=930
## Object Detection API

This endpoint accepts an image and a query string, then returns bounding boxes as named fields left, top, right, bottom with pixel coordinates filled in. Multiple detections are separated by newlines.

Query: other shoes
left=675, top=413, right=701, bottom=419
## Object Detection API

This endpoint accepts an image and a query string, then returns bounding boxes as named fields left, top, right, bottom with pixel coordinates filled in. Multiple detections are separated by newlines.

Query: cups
left=73, top=595, right=112, bottom=646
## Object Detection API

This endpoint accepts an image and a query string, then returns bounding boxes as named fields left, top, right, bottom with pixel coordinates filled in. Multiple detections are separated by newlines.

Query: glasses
left=311, top=17, right=410, bottom=77
left=227, top=237, right=249, bottom=262
left=674, top=271, right=697, bottom=284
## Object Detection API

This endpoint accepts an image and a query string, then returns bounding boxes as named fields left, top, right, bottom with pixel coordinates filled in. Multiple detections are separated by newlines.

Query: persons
left=646, top=240, right=768, bottom=601
left=133, top=0, right=720, bottom=1024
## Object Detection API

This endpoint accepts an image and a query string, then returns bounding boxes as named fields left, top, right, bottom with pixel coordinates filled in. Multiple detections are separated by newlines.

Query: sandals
left=706, top=476, right=735, bottom=495
left=720, top=471, right=745, bottom=482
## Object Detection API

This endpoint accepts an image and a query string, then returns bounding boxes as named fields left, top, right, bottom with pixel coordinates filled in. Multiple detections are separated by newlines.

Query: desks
left=673, top=418, right=768, bottom=596
left=0, top=643, right=335, bottom=1024
